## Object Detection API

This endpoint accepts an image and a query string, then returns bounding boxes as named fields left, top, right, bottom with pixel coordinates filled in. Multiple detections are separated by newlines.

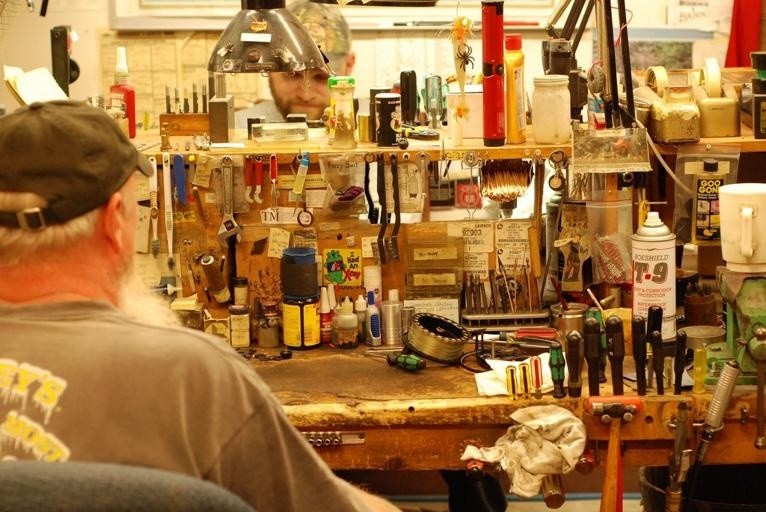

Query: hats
left=1, top=100, right=153, bottom=231
left=286, top=1, right=350, bottom=55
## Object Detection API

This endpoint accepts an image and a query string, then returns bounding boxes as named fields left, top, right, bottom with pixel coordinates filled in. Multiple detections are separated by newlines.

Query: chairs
left=0, top=458, right=253, bottom=511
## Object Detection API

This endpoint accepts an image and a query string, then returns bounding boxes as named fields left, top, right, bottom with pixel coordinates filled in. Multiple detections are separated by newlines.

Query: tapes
left=645, top=65, right=668, bottom=99
left=699, top=57, right=721, bottom=97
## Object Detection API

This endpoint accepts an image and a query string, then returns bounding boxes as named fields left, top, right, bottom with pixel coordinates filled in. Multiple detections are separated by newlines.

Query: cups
left=605, top=307, right=634, bottom=355
left=718, top=181, right=766, bottom=274
left=549, top=302, right=589, bottom=351
left=685, top=295, right=718, bottom=324
left=676, top=325, right=728, bottom=351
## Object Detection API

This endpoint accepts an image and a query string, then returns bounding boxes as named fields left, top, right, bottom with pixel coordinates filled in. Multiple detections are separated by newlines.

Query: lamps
left=198, top=0, right=330, bottom=76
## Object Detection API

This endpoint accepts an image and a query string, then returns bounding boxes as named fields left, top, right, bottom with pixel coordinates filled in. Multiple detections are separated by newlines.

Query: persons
left=234, top=0, right=370, bottom=128
left=1, top=102, right=401, bottom=512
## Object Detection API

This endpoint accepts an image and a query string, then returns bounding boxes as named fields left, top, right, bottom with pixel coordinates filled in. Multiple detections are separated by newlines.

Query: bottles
left=329, top=70, right=447, bottom=150
left=365, top=290, right=382, bottom=346
left=504, top=32, right=527, bottom=144
left=381, top=301, right=404, bottom=345
left=319, top=283, right=338, bottom=341
left=530, top=76, right=572, bottom=146
left=107, top=46, right=136, bottom=141
left=232, top=275, right=249, bottom=306
left=292, top=159, right=309, bottom=195
left=340, top=295, right=359, bottom=347
left=630, top=210, right=681, bottom=341
left=355, top=295, right=367, bottom=340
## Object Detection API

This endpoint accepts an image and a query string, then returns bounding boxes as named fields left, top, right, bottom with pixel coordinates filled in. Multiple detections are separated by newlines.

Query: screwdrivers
left=363, top=351, right=426, bottom=371
left=505, top=306, right=707, bottom=458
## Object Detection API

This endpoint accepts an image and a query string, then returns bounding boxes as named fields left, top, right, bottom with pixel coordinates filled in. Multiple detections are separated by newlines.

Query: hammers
left=583, top=396, right=642, bottom=512
